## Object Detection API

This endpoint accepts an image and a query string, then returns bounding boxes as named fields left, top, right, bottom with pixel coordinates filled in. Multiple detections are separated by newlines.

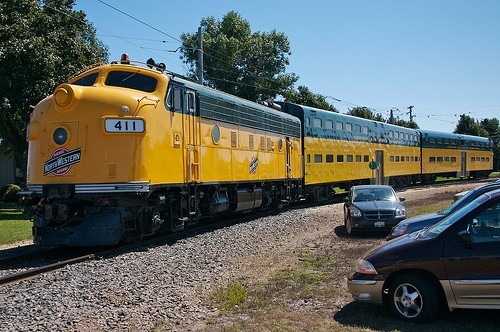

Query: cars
left=342, top=179, right=500, bottom=320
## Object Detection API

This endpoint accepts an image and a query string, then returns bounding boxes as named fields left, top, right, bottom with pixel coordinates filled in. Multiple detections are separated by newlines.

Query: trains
left=25, top=53, right=495, bottom=253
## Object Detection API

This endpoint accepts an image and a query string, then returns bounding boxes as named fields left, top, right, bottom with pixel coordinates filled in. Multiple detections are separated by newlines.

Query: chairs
left=354, top=192, right=377, bottom=202
